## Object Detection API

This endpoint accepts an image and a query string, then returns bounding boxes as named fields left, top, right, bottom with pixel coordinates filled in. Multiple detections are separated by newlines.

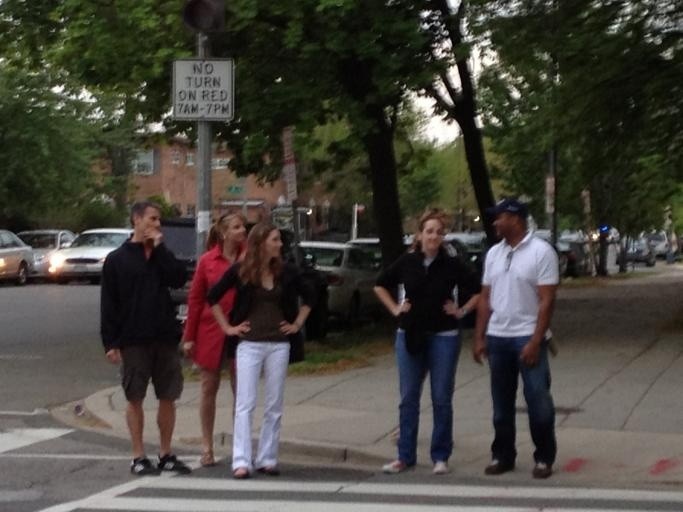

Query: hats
left=486, top=199, right=526, bottom=217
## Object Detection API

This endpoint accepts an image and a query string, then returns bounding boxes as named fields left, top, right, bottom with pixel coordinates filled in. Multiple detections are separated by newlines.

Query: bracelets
left=293, top=321, right=301, bottom=329
left=461, top=306, right=468, bottom=315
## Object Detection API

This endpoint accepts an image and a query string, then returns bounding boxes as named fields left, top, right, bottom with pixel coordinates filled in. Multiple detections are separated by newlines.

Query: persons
left=101, top=200, right=187, bottom=478
left=370, top=210, right=480, bottom=476
left=473, top=200, right=561, bottom=476
left=204, top=221, right=317, bottom=478
left=181, top=214, right=252, bottom=466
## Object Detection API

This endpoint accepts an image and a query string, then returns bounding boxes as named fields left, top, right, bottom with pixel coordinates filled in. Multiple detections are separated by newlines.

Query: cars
left=1, top=225, right=136, bottom=286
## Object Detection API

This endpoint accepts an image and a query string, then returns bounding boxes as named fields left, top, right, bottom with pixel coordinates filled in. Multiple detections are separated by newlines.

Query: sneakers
left=434, top=461, right=449, bottom=474
left=234, top=467, right=279, bottom=478
left=485, top=461, right=514, bottom=475
left=533, top=463, right=550, bottom=478
left=383, top=461, right=407, bottom=474
left=131, top=453, right=213, bottom=475
